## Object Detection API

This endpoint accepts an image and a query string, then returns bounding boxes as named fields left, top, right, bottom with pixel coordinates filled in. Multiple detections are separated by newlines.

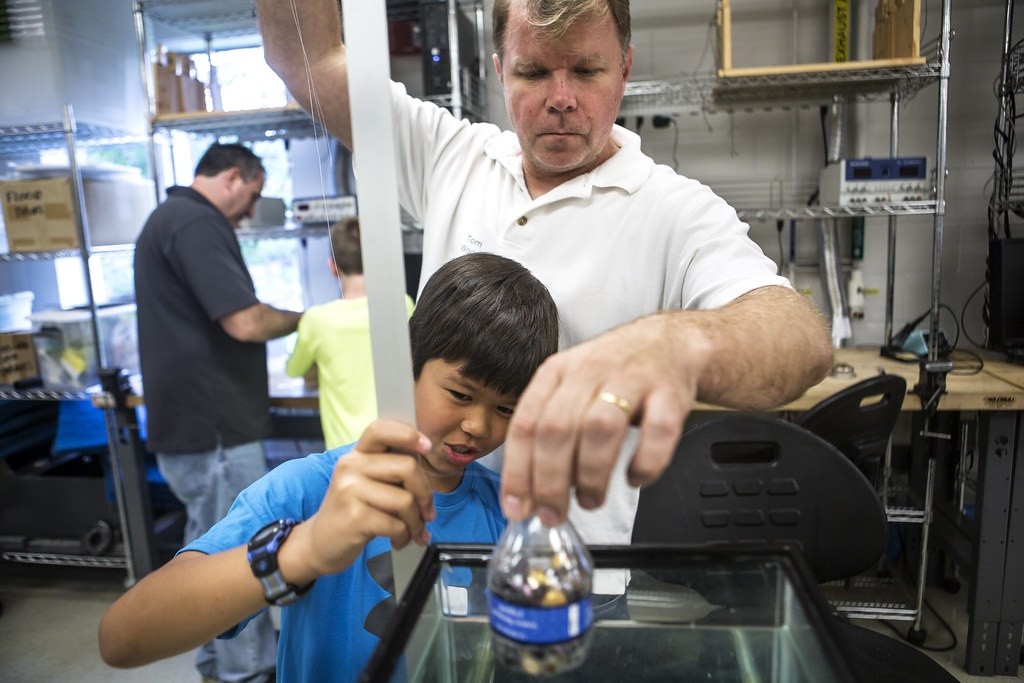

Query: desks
left=86, top=347, right=1024, bottom=676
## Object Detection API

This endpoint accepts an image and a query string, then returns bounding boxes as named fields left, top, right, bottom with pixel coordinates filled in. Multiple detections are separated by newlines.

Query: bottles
left=487, top=508, right=594, bottom=682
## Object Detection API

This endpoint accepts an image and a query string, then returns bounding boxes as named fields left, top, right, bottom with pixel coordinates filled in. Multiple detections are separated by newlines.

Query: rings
left=599, top=392, right=632, bottom=412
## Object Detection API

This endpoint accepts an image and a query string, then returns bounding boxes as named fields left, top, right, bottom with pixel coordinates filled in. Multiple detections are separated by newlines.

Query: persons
left=135, top=141, right=418, bottom=683
left=255, top=0, right=835, bottom=593
left=98, top=252, right=560, bottom=683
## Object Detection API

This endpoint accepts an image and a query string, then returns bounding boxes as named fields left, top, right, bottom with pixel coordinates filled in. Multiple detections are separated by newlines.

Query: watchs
left=247, top=519, right=313, bottom=606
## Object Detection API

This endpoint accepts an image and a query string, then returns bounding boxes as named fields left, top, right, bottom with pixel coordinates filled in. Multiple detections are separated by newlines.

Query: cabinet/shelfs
left=136, top=1, right=485, bottom=247
left=618, top=60, right=951, bottom=391
left=0, top=103, right=189, bottom=588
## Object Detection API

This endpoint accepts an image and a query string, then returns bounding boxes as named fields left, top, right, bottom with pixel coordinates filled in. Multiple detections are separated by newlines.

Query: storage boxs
left=0, top=175, right=158, bottom=252
left=0, top=331, right=40, bottom=386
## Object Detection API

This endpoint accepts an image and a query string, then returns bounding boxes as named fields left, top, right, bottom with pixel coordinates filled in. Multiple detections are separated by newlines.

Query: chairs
left=630, top=376, right=961, bottom=683
